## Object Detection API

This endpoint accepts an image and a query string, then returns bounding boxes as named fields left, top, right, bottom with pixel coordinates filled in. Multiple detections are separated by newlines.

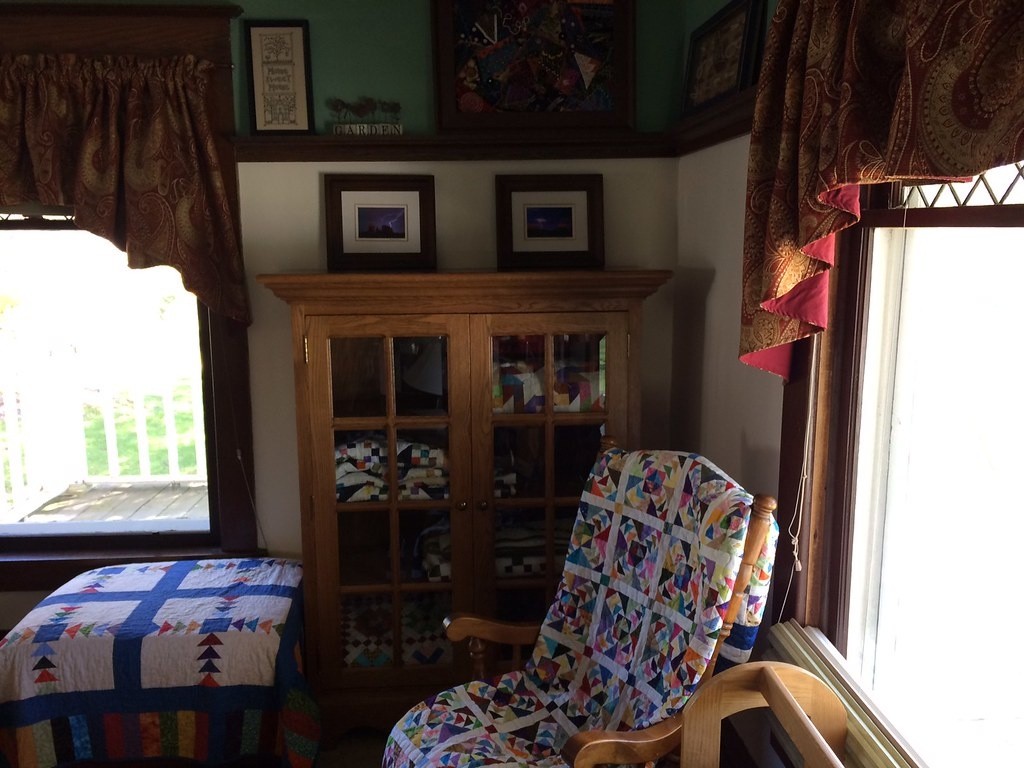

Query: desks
left=0, top=556, right=320, bottom=768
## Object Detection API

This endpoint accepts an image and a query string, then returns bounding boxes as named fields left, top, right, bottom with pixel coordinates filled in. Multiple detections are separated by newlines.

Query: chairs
left=382, top=437, right=781, bottom=768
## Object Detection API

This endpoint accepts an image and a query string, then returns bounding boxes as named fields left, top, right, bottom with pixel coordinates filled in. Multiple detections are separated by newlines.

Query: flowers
left=330, top=97, right=400, bottom=124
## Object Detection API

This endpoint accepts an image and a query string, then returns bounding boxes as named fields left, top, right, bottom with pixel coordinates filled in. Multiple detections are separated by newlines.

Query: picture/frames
left=495, top=174, right=606, bottom=270
left=323, top=173, right=438, bottom=271
left=682, top=0, right=766, bottom=120
left=430, top=0, right=636, bottom=132
left=242, top=18, right=313, bottom=133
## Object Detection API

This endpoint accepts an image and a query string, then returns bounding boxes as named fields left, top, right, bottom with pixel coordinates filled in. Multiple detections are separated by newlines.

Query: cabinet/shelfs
left=258, top=269, right=673, bottom=746
